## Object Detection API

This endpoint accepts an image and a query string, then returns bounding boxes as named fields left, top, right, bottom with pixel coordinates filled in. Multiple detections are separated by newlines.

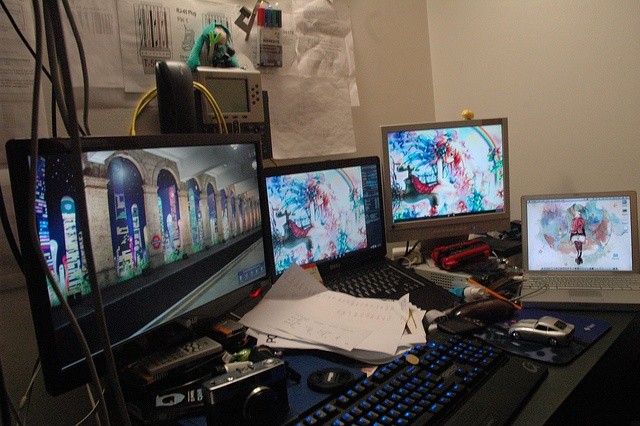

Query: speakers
left=155, top=62, right=194, bottom=132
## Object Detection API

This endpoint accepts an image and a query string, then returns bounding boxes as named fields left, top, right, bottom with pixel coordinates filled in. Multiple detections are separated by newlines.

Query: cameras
left=203, top=357, right=289, bottom=425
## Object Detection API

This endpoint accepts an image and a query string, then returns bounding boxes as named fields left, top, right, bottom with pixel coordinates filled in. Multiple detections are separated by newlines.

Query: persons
left=568, top=204, right=587, bottom=264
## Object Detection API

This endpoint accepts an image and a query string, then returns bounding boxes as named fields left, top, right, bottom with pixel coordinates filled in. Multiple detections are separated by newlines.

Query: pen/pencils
left=465, top=276, right=522, bottom=310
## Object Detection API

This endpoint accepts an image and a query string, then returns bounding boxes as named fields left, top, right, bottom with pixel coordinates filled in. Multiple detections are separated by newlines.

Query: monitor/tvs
left=195, top=66, right=263, bottom=125
left=380, top=117, right=511, bottom=269
left=7, top=133, right=272, bottom=395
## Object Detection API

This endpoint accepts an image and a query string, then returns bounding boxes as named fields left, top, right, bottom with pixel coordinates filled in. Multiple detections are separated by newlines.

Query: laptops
left=263, top=156, right=464, bottom=321
left=519, top=189, right=636, bottom=312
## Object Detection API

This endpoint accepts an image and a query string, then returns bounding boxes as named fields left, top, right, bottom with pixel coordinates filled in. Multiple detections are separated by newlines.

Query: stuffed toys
left=186, top=22, right=238, bottom=70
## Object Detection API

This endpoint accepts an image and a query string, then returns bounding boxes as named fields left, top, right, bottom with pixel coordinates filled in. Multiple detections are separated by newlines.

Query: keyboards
left=279, top=336, right=550, bottom=426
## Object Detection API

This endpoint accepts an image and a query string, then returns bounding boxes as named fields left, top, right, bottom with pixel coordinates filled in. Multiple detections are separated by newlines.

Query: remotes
left=139, top=337, right=223, bottom=376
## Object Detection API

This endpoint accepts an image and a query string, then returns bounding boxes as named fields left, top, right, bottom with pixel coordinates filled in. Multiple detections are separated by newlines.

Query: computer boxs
left=402, top=262, right=511, bottom=298
